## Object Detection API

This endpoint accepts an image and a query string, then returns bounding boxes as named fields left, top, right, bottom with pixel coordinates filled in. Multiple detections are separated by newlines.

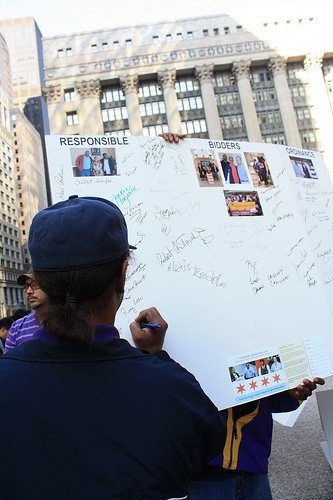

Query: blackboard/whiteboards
left=44, top=134, right=331, bottom=412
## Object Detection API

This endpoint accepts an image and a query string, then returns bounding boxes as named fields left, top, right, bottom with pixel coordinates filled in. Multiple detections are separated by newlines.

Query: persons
left=0, top=195, right=226, bottom=500
left=76, top=150, right=115, bottom=177
left=160, top=133, right=326, bottom=500
left=0, top=269, right=50, bottom=356
left=293, top=159, right=317, bottom=179
left=228, top=355, right=282, bottom=383
left=197, top=154, right=270, bottom=216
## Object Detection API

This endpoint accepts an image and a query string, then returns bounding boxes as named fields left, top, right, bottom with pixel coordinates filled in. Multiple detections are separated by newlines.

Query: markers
left=140, top=323, right=161, bottom=330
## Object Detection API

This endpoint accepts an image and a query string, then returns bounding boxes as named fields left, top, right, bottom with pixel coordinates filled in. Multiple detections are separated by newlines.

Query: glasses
left=23, top=280, right=41, bottom=291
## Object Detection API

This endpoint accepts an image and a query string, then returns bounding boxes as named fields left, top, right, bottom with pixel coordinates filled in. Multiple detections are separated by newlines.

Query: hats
left=16, top=268, right=34, bottom=286
left=27, top=194, right=137, bottom=271
